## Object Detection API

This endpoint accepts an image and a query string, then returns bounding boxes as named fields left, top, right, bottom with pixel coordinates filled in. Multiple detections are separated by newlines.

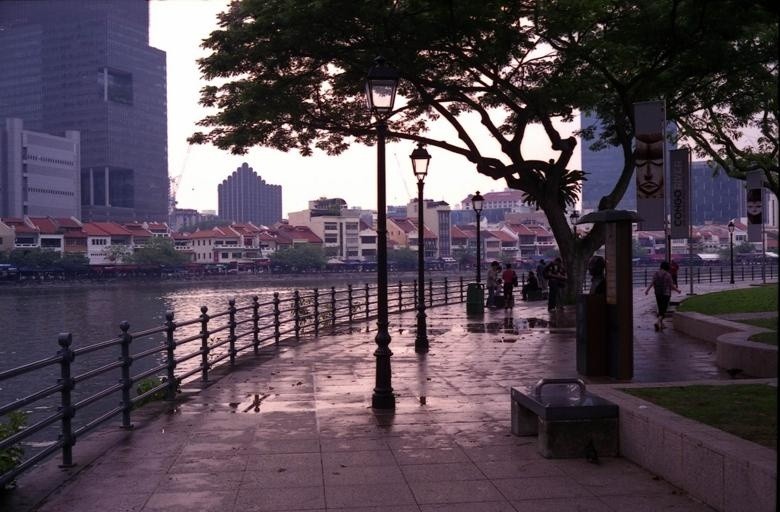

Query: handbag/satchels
left=513, top=270, right=517, bottom=284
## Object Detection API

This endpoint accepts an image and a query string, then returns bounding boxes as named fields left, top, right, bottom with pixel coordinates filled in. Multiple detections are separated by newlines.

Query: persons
left=670, top=260, right=679, bottom=288
left=486, top=258, right=567, bottom=311
left=645, top=262, right=682, bottom=331
left=588, top=256, right=606, bottom=297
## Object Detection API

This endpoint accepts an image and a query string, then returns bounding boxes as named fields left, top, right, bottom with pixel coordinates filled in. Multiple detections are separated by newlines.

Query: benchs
left=492, top=294, right=515, bottom=310
left=528, top=289, right=548, bottom=301
left=509, top=377, right=622, bottom=463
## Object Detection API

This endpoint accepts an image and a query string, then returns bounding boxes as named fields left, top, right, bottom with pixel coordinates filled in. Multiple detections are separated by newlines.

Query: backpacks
left=653, top=271, right=667, bottom=295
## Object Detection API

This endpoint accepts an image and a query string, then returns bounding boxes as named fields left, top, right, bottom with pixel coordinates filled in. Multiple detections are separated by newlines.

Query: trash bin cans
left=467, top=283, right=485, bottom=314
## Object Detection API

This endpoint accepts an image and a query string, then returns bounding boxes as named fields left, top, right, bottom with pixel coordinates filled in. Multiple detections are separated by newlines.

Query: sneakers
left=655, top=321, right=660, bottom=331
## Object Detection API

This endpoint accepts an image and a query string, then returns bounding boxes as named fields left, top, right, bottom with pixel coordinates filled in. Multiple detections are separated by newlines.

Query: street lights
left=663, top=220, right=672, bottom=265
left=727, top=221, right=736, bottom=284
left=362, top=54, right=402, bottom=410
left=409, top=144, right=433, bottom=350
left=471, top=190, right=486, bottom=290
left=569, top=210, right=579, bottom=233
